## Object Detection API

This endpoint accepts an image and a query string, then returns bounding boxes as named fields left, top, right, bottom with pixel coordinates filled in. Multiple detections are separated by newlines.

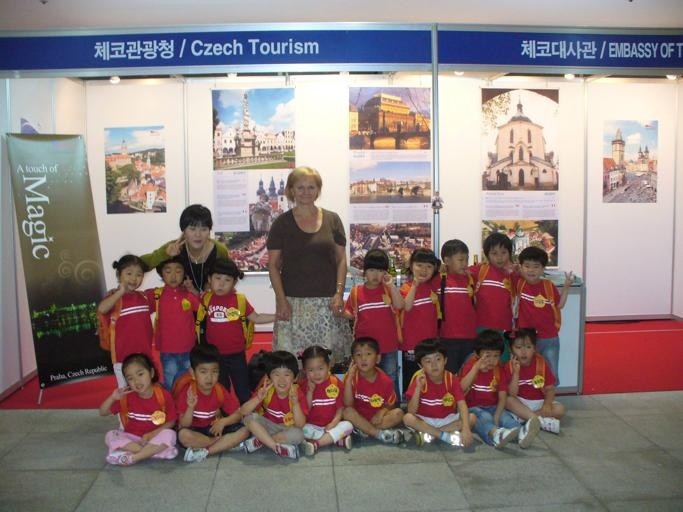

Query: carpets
left=0, top=317, right=681, bottom=412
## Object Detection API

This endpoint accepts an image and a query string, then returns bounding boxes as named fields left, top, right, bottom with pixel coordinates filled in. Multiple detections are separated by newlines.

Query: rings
left=338, top=307, right=342, bottom=312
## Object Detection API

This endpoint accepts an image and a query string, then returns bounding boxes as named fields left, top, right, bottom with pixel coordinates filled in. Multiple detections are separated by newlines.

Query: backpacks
left=98, top=289, right=123, bottom=350
left=204, top=293, right=254, bottom=348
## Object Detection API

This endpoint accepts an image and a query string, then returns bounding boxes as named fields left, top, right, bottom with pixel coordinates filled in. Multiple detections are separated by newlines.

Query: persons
left=241, top=350, right=308, bottom=459
left=98, top=253, right=154, bottom=430
left=332, top=249, right=404, bottom=407
left=98, top=351, right=177, bottom=464
left=503, top=328, right=564, bottom=434
left=342, top=336, right=405, bottom=447
left=398, top=248, right=443, bottom=391
left=433, top=239, right=479, bottom=373
left=460, top=330, right=541, bottom=450
left=444, top=233, right=521, bottom=367
left=475, top=248, right=575, bottom=403
left=139, top=203, right=231, bottom=343
left=266, top=166, right=352, bottom=372
left=183, top=257, right=276, bottom=407
left=173, top=343, right=250, bottom=465
left=297, top=345, right=353, bottom=456
left=402, top=337, right=477, bottom=448
left=108, top=259, right=205, bottom=392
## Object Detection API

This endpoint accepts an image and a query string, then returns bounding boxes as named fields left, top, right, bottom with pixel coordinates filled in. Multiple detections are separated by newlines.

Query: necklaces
left=185, top=241, right=209, bottom=295
left=186, top=244, right=207, bottom=265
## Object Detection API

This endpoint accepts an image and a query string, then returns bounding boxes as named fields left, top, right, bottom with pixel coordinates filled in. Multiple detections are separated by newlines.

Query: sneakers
left=228, top=436, right=263, bottom=454
left=275, top=439, right=319, bottom=459
left=183, top=447, right=209, bottom=463
left=450, top=431, right=464, bottom=447
left=335, top=435, right=352, bottom=449
left=106, top=453, right=133, bottom=466
left=492, top=416, right=560, bottom=449
left=378, top=429, right=433, bottom=447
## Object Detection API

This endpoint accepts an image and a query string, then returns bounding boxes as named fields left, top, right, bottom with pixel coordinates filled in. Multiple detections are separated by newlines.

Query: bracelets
left=335, top=280, right=345, bottom=296
